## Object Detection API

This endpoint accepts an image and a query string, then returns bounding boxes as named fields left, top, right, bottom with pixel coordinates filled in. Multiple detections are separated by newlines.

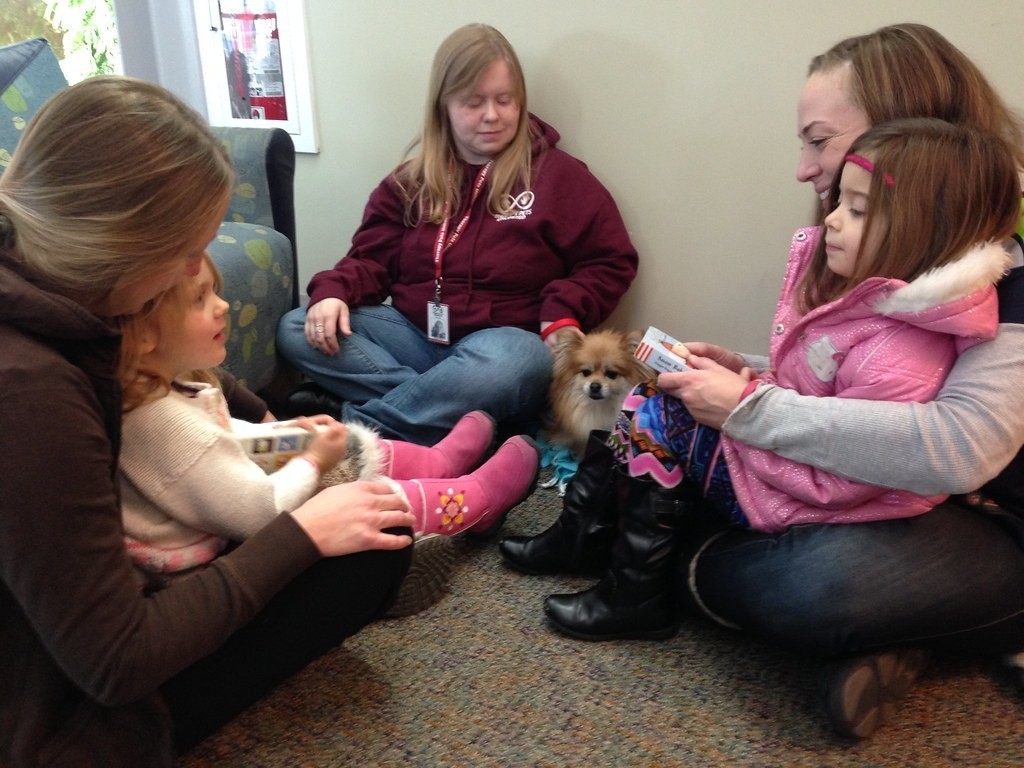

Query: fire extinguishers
left=237, top=3, right=289, bottom=121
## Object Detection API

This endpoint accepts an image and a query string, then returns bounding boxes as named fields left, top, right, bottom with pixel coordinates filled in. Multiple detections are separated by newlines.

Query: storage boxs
left=632, top=325, right=697, bottom=374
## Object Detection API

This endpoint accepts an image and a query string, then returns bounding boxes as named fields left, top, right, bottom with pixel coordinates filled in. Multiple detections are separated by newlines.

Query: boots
left=375, top=434, right=539, bottom=539
left=541, top=467, right=702, bottom=642
left=497, top=430, right=629, bottom=579
left=374, top=411, right=497, bottom=480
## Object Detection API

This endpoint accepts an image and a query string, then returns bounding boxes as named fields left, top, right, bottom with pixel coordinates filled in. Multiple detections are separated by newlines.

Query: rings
left=315, top=324, right=322, bottom=327
left=316, top=330, right=324, bottom=333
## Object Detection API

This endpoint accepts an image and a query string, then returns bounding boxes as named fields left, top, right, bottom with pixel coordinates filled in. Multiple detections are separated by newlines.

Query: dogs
left=541, top=327, right=646, bottom=453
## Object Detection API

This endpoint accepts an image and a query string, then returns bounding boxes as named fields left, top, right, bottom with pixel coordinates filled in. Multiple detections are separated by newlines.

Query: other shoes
left=823, top=643, right=930, bottom=739
left=381, top=533, right=456, bottom=623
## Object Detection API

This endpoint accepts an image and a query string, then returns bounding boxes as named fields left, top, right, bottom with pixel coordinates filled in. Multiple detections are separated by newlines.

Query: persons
left=431, top=321, right=444, bottom=338
left=109, top=252, right=543, bottom=591
left=656, top=23, right=1024, bottom=738
left=273, top=21, right=639, bottom=448
left=0, top=77, right=454, bottom=768
left=498, top=119, right=1024, bottom=642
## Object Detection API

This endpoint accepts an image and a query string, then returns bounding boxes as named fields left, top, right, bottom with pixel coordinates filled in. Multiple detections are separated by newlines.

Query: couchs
left=0, top=35, right=310, bottom=409
